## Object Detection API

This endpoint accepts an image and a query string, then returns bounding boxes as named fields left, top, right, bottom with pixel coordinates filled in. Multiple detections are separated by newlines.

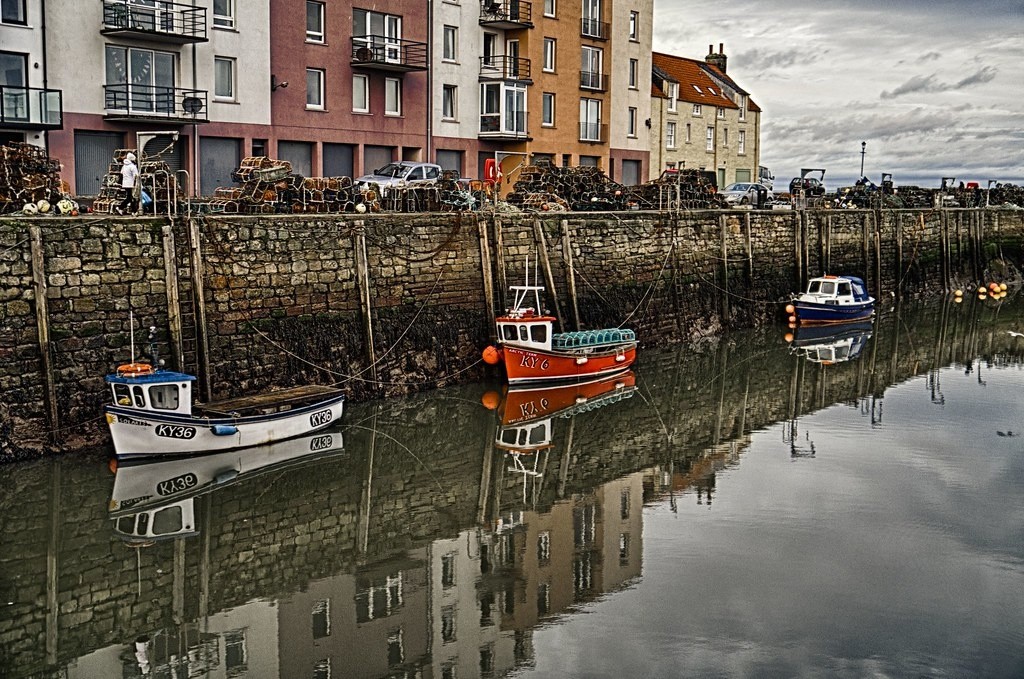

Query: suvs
left=353, top=161, right=442, bottom=200
left=789, top=177, right=825, bottom=197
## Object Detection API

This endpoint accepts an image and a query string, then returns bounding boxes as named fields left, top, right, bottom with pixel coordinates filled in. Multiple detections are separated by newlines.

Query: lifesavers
left=505, top=307, right=536, bottom=317
left=116, top=363, right=152, bottom=371
left=824, top=275, right=837, bottom=278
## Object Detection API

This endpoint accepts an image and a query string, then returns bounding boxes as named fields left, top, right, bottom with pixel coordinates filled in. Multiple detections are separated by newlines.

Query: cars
left=716, top=182, right=775, bottom=209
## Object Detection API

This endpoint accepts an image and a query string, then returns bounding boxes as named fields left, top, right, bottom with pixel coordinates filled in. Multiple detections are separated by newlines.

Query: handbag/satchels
left=141, top=191, right=152, bottom=205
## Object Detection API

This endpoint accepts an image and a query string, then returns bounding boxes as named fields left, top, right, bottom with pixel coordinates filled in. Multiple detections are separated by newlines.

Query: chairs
left=485, top=2, right=503, bottom=20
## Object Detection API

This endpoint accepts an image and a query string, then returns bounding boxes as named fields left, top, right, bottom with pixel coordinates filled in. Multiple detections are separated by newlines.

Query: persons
left=115, top=153, right=140, bottom=217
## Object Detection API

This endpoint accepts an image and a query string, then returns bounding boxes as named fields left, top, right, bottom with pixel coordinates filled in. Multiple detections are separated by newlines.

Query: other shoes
left=116, top=207, right=124, bottom=216
left=132, top=212, right=138, bottom=217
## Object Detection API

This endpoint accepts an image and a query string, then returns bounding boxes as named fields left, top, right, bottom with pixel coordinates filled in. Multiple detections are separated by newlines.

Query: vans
left=758, top=165, right=775, bottom=191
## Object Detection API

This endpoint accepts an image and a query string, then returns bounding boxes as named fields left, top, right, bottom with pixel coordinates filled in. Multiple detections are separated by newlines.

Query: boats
left=785, top=271, right=876, bottom=324
left=101, top=311, right=350, bottom=462
left=481, top=369, right=638, bottom=511
left=784, top=318, right=874, bottom=368
left=482, top=245, right=640, bottom=385
left=104, top=419, right=352, bottom=598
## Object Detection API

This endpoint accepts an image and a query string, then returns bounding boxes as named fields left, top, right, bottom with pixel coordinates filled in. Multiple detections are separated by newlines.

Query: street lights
left=860, top=140, right=866, bottom=176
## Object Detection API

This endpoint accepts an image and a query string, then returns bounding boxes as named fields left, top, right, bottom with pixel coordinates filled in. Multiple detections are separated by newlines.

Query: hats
left=127, top=153, right=136, bottom=161
